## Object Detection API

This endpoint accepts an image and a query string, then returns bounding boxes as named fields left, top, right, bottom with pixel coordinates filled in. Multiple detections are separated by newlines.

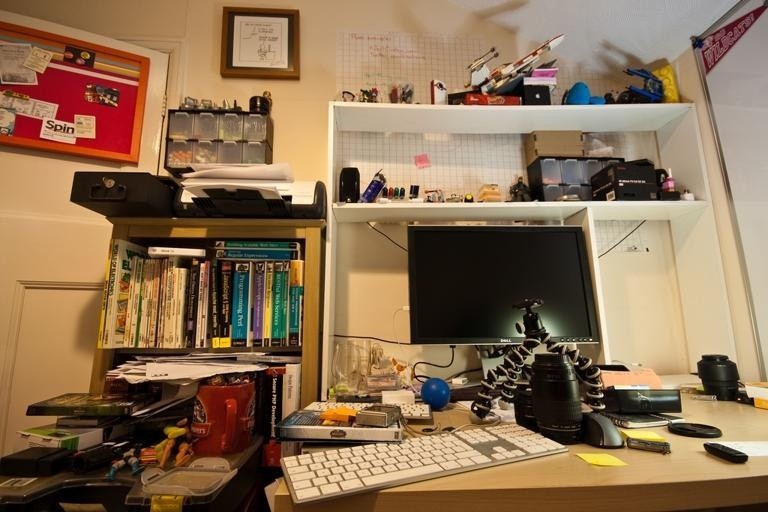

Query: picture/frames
left=219, top=7, right=300, bottom=80
left=0, top=20, right=151, bottom=166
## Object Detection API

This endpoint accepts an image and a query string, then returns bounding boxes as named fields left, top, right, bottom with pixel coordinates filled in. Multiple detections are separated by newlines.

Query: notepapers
left=620, top=429, right=663, bottom=443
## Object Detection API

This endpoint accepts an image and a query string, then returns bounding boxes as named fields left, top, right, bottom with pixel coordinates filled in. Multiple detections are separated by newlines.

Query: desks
left=272, top=379, right=768, bottom=511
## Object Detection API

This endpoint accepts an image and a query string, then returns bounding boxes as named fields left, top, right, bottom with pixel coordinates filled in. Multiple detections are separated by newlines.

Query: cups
left=187, top=379, right=257, bottom=457
left=249, top=95, right=270, bottom=114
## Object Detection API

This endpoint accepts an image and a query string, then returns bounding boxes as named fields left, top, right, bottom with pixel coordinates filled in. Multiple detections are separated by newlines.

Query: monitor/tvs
left=406, top=223, right=602, bottom=347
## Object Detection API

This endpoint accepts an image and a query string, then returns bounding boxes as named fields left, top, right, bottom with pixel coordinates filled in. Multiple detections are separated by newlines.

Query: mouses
left=582, top=411, right=626, bottom=450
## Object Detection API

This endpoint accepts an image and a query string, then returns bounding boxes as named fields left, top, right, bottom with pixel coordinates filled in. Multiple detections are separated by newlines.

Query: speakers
left=338, top=167, right=360, bottom=203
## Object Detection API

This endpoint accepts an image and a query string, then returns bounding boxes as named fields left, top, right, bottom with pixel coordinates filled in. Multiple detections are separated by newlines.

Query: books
left=618, top=424, right=670, bottom=456
left=24, top=390, right=155, bottom=417
left=593, top=353, right=665, bottom=390
left=94, top=238, right=305, bottom=349
left=281, top=364, right=303, bottom=461
left=261, top=365, right=284, bottom=469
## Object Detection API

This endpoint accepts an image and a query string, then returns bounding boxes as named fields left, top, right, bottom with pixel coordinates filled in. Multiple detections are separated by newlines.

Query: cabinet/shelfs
left=91, top=216, right=325, bottom=453
left=320, top=99, right=740, bottom=406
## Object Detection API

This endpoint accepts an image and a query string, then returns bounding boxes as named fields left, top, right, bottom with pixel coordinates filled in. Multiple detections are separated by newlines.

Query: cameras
left=514, top=352, right=584, bottom=445
left=356, top=403, right=403, bottom=427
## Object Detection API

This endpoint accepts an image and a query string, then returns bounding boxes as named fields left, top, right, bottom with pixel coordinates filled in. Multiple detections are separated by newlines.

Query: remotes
left=704, top=440, right=750, bottom=463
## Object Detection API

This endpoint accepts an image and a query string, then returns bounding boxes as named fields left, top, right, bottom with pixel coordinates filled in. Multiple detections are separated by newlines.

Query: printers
left=175, top=161, right=326, bottom=219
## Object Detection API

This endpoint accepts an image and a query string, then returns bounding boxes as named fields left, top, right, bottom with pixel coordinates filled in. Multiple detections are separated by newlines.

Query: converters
left=380, top=390, right=416, bottom=405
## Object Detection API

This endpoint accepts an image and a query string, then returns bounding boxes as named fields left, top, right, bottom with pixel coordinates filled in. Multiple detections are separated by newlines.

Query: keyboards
left=303, top=399, right=436, bottom=428
left=278, top=423, right=570, bottom=507
